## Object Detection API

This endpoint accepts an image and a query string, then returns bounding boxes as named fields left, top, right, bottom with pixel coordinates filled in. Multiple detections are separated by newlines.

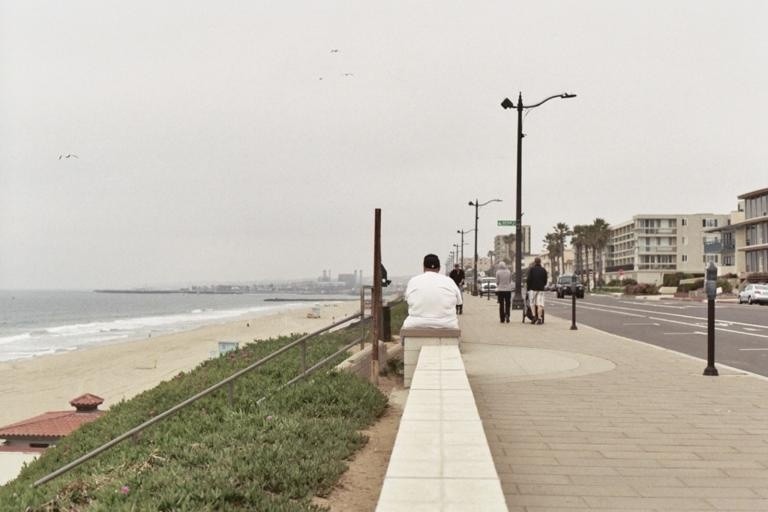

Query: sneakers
left=501, top=314, right=510, bottom=323
left=531, top=315, right=542, bottom=324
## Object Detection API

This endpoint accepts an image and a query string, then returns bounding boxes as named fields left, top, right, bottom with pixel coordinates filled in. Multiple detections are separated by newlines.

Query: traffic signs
left=497, top=220, right=515, bottom=226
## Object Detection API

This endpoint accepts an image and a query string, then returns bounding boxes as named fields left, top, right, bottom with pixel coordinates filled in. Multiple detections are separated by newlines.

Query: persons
left=494, top=261, right=513, bottom=322
left=525, top=257, right=547, bottom=324
left=448, top=263, right=465, bottom=316
left=400, top=253, right=464, bottom=346
left=523, top=293, right=544, bottom=324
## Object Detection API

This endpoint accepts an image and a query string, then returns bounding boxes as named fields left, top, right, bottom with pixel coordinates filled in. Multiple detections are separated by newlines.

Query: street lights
left=468, top=198, right=502, bottom=296
left=457, top=228, right=478, bottom=269
left=501, top=91, right=576, bottom=309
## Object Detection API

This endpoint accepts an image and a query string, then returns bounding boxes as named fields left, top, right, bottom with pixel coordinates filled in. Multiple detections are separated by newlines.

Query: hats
left=424, top=255, right=440, bottom=268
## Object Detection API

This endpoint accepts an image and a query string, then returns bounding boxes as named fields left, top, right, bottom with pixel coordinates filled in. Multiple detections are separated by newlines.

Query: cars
left=476, top=277, right=496, bottom=296
left=555, top=275, right=583, bottom=298
left=738, top=284, right=767, bottom=305
